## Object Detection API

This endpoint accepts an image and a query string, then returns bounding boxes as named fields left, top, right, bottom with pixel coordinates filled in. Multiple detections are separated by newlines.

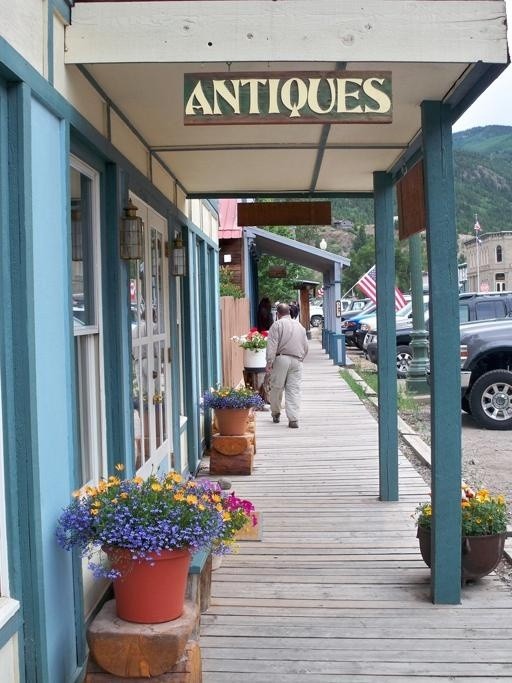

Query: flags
left=474, top=216, right=483, bottom=234
left=357, top=266, right=408, bottom=313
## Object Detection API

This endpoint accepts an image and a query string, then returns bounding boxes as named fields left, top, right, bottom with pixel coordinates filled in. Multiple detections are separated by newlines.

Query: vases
left=101, top=544, right=191, bottom=622
left=242, top=348, right=267, bottom=368
left=213, top=407, right=249, bottom=435
left=416, top=524, right=512, bottom=587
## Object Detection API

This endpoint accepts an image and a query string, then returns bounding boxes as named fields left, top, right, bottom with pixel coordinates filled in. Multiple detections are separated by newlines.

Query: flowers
left=410, top=480, right=512, bottom=534
left=230, top=326, right=269, bottom=349
left=53, top=471, right=256, bottom=584
left=199, top=384, right=268, bottom=411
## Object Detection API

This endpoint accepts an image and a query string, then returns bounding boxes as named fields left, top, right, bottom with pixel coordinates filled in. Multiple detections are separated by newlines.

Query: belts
left=275, top=352, right=300, bottom=359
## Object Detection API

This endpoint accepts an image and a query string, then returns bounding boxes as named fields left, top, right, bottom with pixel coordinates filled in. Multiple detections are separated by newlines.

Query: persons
left=289, top=299, right=300, bottom=319
left=259, top=296, right=274, bottom=331
left=265, top=303, right=308, bottom=427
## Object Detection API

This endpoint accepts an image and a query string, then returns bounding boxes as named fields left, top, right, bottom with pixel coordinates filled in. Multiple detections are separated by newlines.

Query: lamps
left=320, top=238, right=327, bottom=249
left=72, top=208, right=86, bottom=260
left=120, top=194, right=143, bottom=262
left=165, top=231, right=187, bottom=277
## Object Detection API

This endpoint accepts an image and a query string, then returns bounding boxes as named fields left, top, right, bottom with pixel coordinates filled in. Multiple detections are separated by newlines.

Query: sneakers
left=271, top=411, right=300, bottom=428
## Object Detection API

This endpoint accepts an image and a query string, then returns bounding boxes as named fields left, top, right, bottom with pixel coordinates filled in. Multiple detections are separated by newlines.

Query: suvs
left=305, top=286, right=512, bottom=376
left=424, top=313, right=512, bottom=431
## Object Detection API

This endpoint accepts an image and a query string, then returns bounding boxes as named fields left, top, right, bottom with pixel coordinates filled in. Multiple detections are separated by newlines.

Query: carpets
left=236, top=510, right=264, bottom=541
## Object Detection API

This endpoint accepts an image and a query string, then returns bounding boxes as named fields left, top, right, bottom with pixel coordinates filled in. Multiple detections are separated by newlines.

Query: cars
left=70, top=298, right=160, bottom=364
